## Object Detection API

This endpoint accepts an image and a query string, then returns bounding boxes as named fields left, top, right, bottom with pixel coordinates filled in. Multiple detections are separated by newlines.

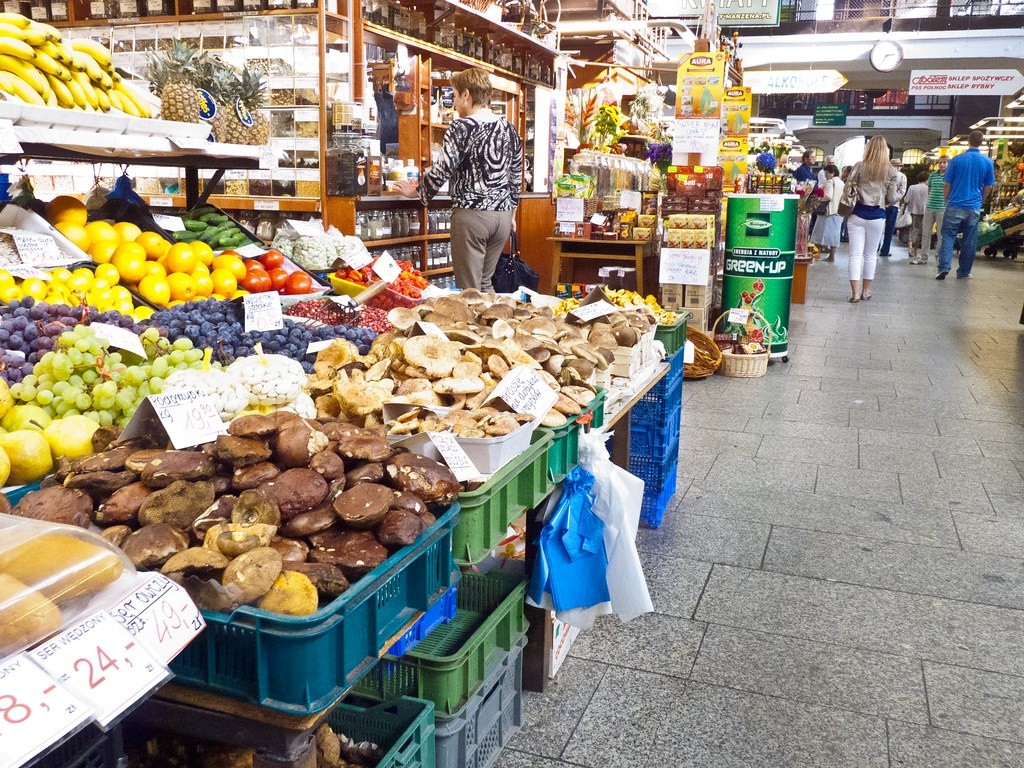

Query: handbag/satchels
left=837, top=162, right=861, bottom=217
left=812, top=200, right=830, bottom=216
left=895, top=202, right=912, bottom=227
left=491, top=229, right=540, bottom=294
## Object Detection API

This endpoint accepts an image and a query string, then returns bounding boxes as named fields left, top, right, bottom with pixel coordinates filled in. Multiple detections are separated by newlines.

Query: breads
left=0, top=527, right=124, bottom=661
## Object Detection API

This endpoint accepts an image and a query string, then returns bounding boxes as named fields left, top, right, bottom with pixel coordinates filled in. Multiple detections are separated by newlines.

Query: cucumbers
left=167, top=206, right=263, bottom=251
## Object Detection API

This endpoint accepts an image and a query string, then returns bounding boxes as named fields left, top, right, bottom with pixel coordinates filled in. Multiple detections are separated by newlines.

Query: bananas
left=0, top=13, right=153, bottom=117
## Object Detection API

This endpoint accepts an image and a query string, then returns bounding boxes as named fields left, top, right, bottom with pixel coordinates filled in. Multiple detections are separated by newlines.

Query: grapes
left=0, top=297, right=375, bottom=428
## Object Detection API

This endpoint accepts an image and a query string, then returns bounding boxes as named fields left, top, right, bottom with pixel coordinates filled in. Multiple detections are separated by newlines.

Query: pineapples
left=143, top=37, right=270, bottom=146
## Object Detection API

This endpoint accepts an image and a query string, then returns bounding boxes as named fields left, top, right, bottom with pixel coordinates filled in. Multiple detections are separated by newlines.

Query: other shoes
left=910, top=259, right=926, bottom=264
left=879, top=253, right=892, bottom=256
left=822, top=256, right=835, bottom=262
left=958, top=273, right=973, bottom=279
left=935, top=270, right=949, bottom=281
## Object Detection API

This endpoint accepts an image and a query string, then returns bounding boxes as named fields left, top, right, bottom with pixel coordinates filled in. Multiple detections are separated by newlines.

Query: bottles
left=220, top=153, right=458, bottom=294
left=2, top=0, right=345, bottom=17
left=359, top=0, right=557, bottom=87
left=991, top=168, right=1018, bottom=212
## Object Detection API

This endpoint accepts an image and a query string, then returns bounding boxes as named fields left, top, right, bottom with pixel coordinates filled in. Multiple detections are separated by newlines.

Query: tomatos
left=218, top=247, right=312, bottom=295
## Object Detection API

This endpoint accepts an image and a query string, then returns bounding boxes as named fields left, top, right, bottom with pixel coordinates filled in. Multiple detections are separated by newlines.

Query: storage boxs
left=632, top=214, right=656, bottom=242
left=162, top=308, right=690, bottom=767
left=661, top=273, right=713, bottom=334
left=661, top=165, right=724, bottom=248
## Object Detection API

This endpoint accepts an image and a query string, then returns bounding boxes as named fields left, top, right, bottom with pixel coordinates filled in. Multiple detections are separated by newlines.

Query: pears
left=0, top=377, right=102, bottom=489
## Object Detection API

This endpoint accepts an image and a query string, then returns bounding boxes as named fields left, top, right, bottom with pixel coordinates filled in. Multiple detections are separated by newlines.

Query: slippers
left=861, top=293, right=872, bottom=300
left=850, top=296, right=861, bottom=303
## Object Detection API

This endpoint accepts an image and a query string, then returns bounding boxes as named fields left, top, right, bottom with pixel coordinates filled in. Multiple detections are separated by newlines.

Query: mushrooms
left=41, top=412, right=461, bottom=618
left=0, top=490, right=12, bottom=515
left=306, top=287, right=658, bottom=439
left=9, top=484, right=95, bottom=529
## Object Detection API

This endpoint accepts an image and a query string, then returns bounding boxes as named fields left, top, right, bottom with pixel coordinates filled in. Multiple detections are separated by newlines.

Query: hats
left=890, top=159, right=902, bottom=165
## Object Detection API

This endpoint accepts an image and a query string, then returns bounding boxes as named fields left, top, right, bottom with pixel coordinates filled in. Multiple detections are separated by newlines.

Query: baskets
left=583, top=198, right=597, bottom=217
left=711, top=309, right=772, bottom=378
left=5, top=311, right=690, bottom=768
left=683, top=325, right=723, bottom=378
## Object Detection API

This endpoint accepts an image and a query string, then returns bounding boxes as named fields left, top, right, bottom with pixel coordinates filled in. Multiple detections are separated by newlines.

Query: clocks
left=869, top=40, right=903, bottom=74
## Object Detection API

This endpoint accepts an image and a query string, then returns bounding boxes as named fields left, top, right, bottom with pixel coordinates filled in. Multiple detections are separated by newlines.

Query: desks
left=545, top=236, right=652, bottom=298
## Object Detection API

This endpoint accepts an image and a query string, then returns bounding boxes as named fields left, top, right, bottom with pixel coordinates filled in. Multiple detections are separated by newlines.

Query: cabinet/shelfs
left=0, top=1, right=559, bottom=288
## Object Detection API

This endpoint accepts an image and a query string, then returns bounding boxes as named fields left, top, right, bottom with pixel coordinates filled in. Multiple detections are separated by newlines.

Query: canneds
left=356, top=208, right=457, bottom=291
left=361, top=0, right=552, bottom=84
left=0, top=0, right=319, bottom=22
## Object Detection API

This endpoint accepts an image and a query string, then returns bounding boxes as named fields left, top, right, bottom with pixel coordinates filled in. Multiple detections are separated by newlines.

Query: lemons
left=0, top=194, right=247, bottom=316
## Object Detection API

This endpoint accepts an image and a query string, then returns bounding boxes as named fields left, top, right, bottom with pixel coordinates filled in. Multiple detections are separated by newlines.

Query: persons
left=877, top=158, right=907, bottom=256
left=902, top=171, right=929, bottom=256
left=936, top=131, right=995, bottom=281
left=794, top=150, right=819, bottom=189
left=840, top=135, right=898, bottom=303
left=393, top=67, right=523, bottom=292
left=910, top=155, right=950, bottom=266
left=840, top=166, right=852, bottom=241
left=809, top=155, right=835, bottom=234
left=811, top=165, right=845, bottom=262
